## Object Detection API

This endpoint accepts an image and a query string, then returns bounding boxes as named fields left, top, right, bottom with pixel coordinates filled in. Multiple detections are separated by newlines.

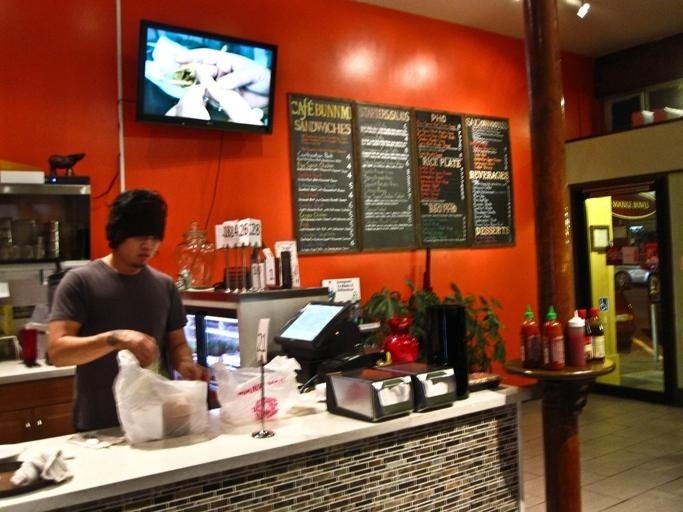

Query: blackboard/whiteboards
left=287, top=92, right=516, bottom=257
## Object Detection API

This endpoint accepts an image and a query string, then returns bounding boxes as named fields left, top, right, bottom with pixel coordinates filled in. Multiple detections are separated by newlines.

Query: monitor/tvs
left=135, top=17, right=277, bottom=135
left=272, top=299, right=351, bottom=348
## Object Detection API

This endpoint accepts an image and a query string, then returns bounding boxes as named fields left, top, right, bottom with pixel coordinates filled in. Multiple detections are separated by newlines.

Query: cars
left=615, top=265, right=653, bottom=336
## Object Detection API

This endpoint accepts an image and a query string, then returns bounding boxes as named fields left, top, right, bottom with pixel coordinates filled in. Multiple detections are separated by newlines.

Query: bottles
left=521, top=303, right=606, bottom=370
left=384, top=317, right=418, bottom=364
left=176, top=221, right=216, bottom=289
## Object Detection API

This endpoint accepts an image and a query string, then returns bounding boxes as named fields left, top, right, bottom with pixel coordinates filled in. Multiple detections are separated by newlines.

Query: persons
left=166, top=47, right=271, bottom=125
left=42, top=189, right=207, bottom=429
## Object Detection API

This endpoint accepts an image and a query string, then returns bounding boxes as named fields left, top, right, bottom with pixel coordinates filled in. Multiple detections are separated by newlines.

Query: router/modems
left=50, top=176, right=91, bottom=184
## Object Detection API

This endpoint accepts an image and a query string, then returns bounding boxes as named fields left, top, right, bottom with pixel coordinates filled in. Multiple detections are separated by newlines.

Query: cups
left=19, top=329, right=38, bottom=365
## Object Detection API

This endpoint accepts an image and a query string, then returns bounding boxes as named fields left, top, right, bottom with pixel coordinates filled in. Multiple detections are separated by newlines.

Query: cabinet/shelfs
left=172, top=288, right=330, bottom=410
left=0, top=374, right=76, bottom=446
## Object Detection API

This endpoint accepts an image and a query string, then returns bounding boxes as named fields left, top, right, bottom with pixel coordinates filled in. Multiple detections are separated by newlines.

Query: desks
left=507, top=356, right=615, bottom=510
